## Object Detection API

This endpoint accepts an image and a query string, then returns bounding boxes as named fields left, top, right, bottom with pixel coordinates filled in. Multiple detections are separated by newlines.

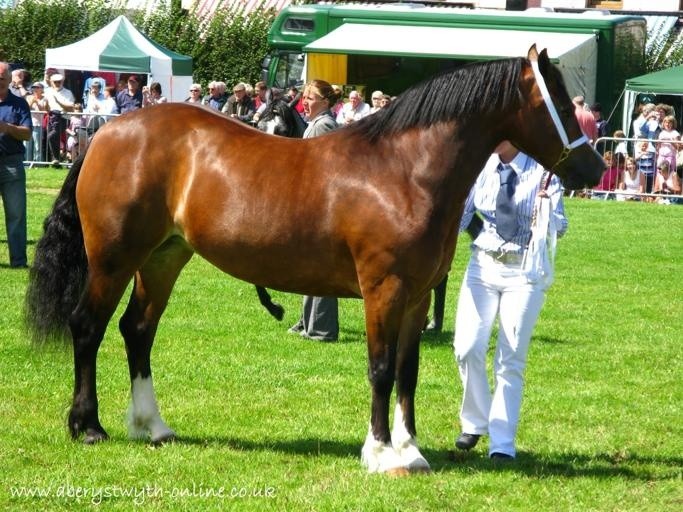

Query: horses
left=21, top=41, right=605, bottom=478
left=254, top=86, right=448, bottom=333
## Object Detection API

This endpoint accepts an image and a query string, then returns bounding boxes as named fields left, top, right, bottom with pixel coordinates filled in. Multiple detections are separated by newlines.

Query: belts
left=485, top=249, right=522, bottom=262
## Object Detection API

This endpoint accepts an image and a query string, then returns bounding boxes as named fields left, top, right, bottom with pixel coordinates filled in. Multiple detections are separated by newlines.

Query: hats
left=127, top=75, right=139, bottom=83
left=233, top=84, right=245, bottom=91
left=31, top=68, right=65, bottom=88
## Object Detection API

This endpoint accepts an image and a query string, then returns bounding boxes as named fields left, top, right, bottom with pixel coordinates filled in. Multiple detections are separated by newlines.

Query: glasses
left=190, top=90, right=199, bottom=92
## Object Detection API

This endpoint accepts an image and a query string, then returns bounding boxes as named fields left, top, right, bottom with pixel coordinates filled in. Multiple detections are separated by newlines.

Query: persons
left=1, top=60, right=683, bottom=462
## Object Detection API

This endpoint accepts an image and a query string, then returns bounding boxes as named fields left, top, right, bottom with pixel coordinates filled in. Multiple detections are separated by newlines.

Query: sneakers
left=491, top=452, right=514, bottom=464
left=455, top=433, right=482, bottom=451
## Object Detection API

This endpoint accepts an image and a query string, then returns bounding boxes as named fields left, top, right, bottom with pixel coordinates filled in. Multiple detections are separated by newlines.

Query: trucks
left=261, top=4, right=647, bottom=152
